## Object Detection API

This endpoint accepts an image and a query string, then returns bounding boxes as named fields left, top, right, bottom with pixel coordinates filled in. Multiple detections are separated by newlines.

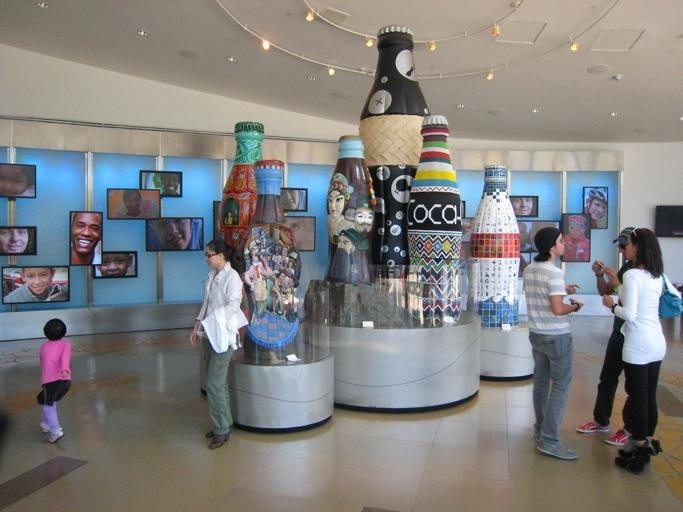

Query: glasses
left=632, top=228, right=639, bottom=241
left=205, top=253, right=220, bottom=257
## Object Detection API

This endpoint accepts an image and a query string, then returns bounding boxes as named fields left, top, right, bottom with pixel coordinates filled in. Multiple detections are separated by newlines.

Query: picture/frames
left=93, top=251, right=138, bottom=278
left=139, top=170, right=183, bottom=198
left=0, top=162, right=36, bottom=199
left=508, top=195, right=539, bottom=217
left=460, top=200, right=467, bottom=218
left=145, top=217, right=204, bottom=251
left=583, top=187, right=608, bottom=229
left=107, top=188, right=161, bottom=221
left=1, top=265, right=70, bottom=304
left=562, top=212, right=591, bottom=262
left=0, top=226, right=37, bottom=256
left=517, top=220, right=560, bottom=253
left=287, top=215, right=317, bottom=253
left=279, top=188, right=308, bottom=212
left=462, top=218, right=476, bottom=243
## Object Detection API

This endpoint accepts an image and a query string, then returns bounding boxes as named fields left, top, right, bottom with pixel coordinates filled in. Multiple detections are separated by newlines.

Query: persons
left=511, top=196, right=533, bottom=217
left=577, top=227, right=640, bottom=445
left=522, top=226, right=584, bottom=460
left=153, top=219, right=193, bottom=250
left=327, top=173, right=354, bottom=245
left=0, top=164, right=33, bottom=197
left=225, top=211, right=234, bottom=226
left=564, top=215, right=590, bottom=260
left=116, top=189, right=150, bottom=218
left=190, top=238, right=243, bottom=450
left=330, top=228, right=360, bottom=282
left=244, top=228, right=295, bottom=319
left=98, top=252, right=133, bottom=275
left=519, top=221, right=528, bottom=249
left=37, top=319, right=71, bottom=443
left=161, top=172, right=180, bottom=196
left=280, top=189, right=300, bottom=209
left=585, top=189, right=608, bottom=228
left=604, top=229, right=682, bottom=473
left=290, top=223, right=302, bottom=249
left=354, top=208, right=374, bottom=234
left=3, top=268, right=68, bottom=303
left=346, top=200, right=357, bottom=222
left=1, top=228, right=29, bottom=254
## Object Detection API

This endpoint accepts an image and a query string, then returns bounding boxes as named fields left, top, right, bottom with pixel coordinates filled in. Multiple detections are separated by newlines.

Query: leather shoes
left=209, top=433, right=230, bottom=449
left=41, top=425, right=50, bottom=434
left=48, top=431, right=63, bottom=444
left=206, top=425, right=233, bottom=437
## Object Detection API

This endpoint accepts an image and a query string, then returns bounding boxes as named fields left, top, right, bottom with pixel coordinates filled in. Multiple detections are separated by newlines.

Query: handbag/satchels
left=658, top=274, right=683, bottom=319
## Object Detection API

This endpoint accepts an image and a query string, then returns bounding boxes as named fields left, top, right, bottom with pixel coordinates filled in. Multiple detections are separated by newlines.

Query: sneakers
left=534, top=431, right=544, bottom=442
left=537, top=442, right=578, bottom=460
left=605, top=429, right=629, bottom=445
left=575, top=422, right=610, bottom=432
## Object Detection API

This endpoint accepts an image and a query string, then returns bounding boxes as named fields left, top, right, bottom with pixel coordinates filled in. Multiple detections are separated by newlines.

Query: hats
left=340, top=229, right=361, bottom=247
left=568, top=216, right=586, bottom=225
left=356, top=195, right=372, bottom=209
left=535, top=227, right=560, bottom=262
left=328, top=174, right=353, bottom=199
left=612, top=227, right=636, bottom=245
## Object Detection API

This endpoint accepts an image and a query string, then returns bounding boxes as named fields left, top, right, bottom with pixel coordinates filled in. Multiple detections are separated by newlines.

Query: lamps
left=306, top=10, right=315, bottom=21
left=491, top=25, right=500, bottom=38
left=326, top=68, right=335, bottom=76
left=366, top=38, right=373, bottom=48
left=486, top=70, right=492, bottom=80
left=262, top=42, right=270, bottom=51
left=427, top=41, right=435, bottom=50
left=570, top=41, right=579, bottom=53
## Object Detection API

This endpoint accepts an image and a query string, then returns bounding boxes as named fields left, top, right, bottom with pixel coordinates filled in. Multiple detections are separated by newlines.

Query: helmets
left=585, top=188, right=607, bottom=219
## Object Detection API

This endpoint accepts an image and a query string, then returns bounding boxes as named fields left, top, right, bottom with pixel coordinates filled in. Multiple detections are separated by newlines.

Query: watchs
left=573, top=301, right=580, bottom=310
left=610, top=304, right=617, bottom=313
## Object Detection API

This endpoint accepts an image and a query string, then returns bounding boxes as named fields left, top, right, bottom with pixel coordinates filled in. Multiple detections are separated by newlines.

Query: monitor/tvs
left=653, top=204, right=683, bottom=238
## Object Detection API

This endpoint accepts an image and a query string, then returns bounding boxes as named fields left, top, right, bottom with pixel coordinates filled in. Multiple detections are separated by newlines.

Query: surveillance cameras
left=613, top=74, right=623, bottom=80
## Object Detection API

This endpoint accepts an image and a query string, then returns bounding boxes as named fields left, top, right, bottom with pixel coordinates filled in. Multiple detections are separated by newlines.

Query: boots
left=615, top=440, right=652, bottom=474
left=618, top=440, right=663, bottom=457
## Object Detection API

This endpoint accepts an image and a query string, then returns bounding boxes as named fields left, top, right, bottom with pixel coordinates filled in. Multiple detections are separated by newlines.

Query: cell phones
left=598, top=262, right=603, bottom=265
